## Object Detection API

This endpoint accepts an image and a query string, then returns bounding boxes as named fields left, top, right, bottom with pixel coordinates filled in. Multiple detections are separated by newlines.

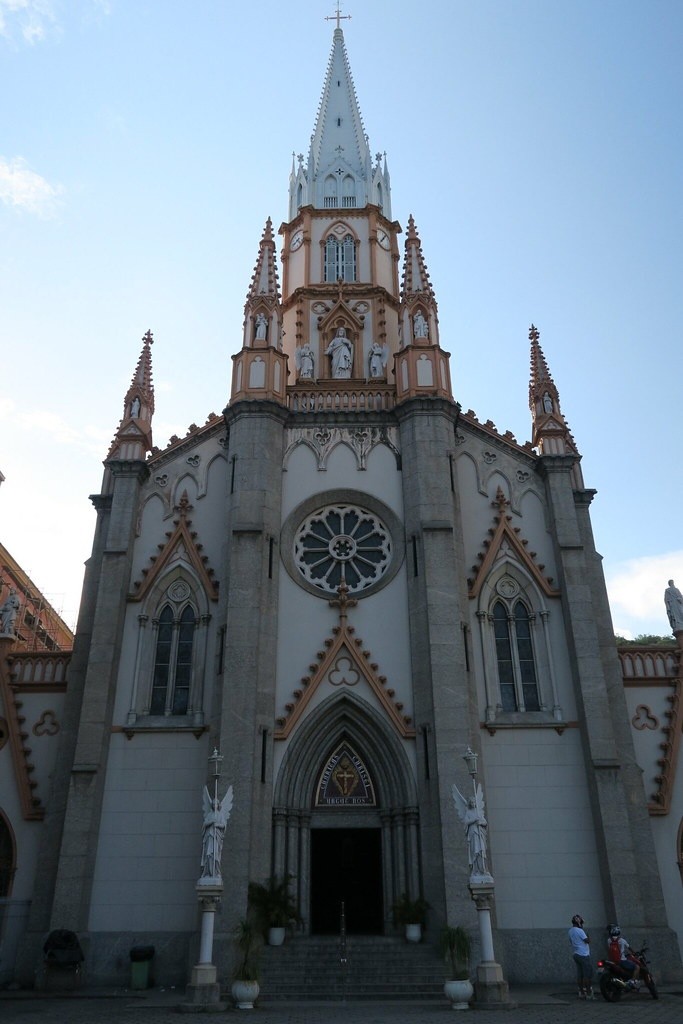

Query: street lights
left=461, top=745, right=493, bottom=884
left=198, top=746, right=225, bottom=886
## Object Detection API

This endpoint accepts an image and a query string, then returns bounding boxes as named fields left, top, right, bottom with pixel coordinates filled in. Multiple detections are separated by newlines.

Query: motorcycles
left=596, top=940, right=658, bottom=1002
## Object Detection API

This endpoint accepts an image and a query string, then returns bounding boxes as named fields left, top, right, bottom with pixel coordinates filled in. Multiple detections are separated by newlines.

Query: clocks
left=377, top=229, right=391, bottom=250
left=290, top=231, right=303, bottom=250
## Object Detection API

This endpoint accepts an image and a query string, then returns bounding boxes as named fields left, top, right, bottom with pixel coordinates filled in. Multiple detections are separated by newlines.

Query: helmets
left=572, top=915, right=584, bottom=928
left=606, top=923, right=620, bottom=936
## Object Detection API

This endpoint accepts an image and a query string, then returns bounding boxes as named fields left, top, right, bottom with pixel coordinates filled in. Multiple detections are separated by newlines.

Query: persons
left=328, top=327, right=353, bottom=379
left=664, top=579, right=683, bottom=631
left=0, top=588, right=21, bottom=634
left=199, top=785, right=233, bottom=878
left=568, top=915, right=596, bottom=999
left=451, top=783, right=491, bottom=876
left=369, top=343, right=383, bottom=377
left=605, top=922, right=640, bottom=986
left=413, top=310, right=427, bottom=337
left=256, top=313, right=268, bottom=339
left=300, top=343, right=313, bottom=377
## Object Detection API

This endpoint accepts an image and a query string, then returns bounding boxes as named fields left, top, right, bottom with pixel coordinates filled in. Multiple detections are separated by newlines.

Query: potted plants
left=388, top=894, right=434, bottom=944
left=250, top=874, right=306, bottom=947
left=432, top=927, right=477, bottom=1010
left=229, top=918, right=260, bottom=1009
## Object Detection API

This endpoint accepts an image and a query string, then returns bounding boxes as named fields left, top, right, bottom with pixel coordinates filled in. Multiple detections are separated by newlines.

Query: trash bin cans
left=128, top=944, right=151, bottom=991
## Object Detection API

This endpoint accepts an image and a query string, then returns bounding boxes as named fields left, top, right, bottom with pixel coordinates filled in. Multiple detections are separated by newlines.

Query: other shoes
left=579, top=993, right=586, bottom=998
left=586, top=996, right=597, bottom=1000
left=629, top=979, right=641, bottom=989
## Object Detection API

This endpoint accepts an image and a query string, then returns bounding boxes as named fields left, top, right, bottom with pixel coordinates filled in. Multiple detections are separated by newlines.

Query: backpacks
left=608, top=937, right=621, bottom=964
